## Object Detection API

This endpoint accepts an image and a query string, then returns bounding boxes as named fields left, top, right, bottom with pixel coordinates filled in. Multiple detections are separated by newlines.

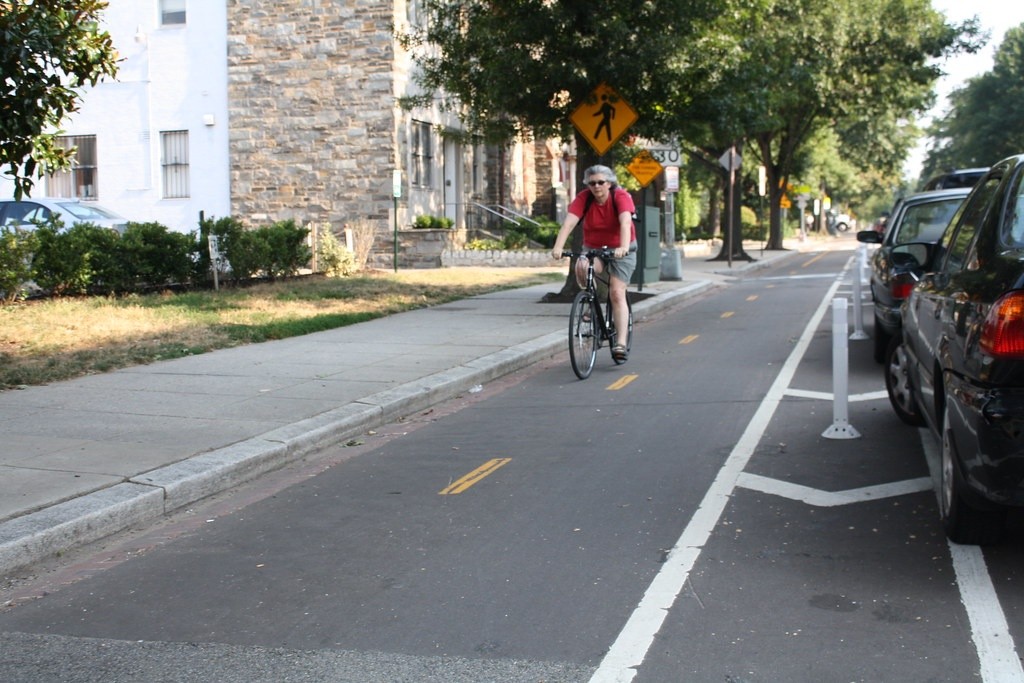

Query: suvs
left=883, top=154, right=1024, bottom=545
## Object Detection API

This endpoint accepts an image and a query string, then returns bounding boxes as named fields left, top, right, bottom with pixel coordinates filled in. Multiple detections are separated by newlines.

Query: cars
left=0, top=199, right=135, bottom=289
left=808, top=212, right=854, bottom=232
left=858, top=187, right=992, bottom=354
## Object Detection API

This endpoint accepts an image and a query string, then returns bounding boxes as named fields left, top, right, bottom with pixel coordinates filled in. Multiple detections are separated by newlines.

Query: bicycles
left=561, top=248, right=634, bottom=380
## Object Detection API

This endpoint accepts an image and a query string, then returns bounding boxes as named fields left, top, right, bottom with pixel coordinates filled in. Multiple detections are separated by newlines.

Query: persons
left=875, top=211, right=892, bottom=235
left=552, top=165, right=639, bottom=355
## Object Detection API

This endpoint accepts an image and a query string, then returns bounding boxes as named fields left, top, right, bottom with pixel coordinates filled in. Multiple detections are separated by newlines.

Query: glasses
left=588, top=180, right=607, bottom=186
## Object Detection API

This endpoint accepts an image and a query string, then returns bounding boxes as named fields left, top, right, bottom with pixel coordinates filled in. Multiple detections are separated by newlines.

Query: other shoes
left=582, top=311, right=592, bottom=322
left=611, top=344, right=627, bottom=355
